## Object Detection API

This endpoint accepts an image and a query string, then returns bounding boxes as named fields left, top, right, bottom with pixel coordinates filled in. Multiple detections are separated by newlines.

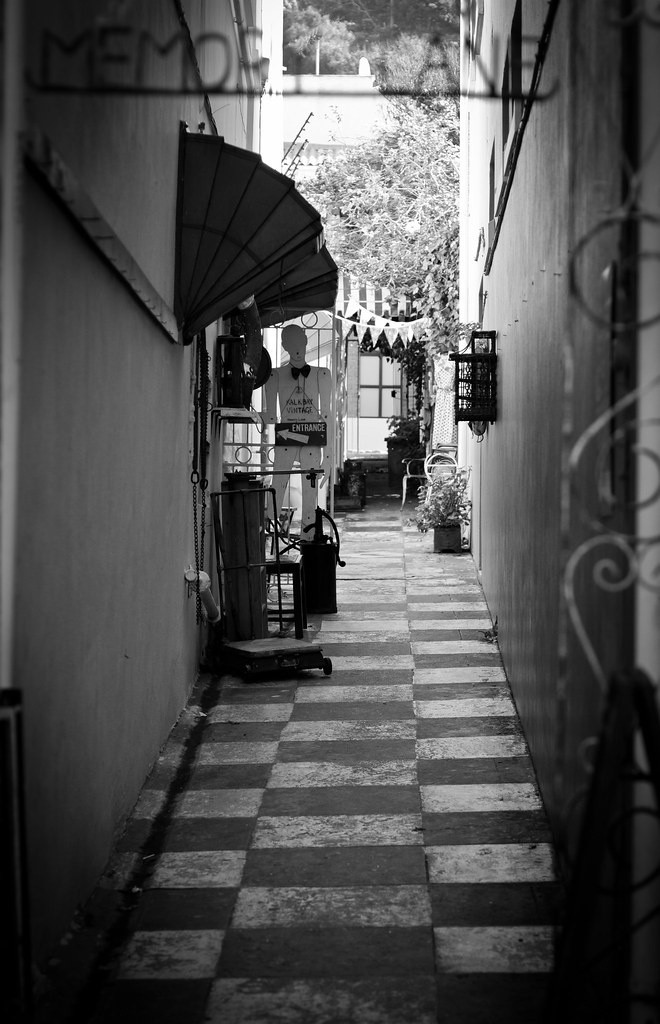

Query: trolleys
left=212, top=486, right=333, bottom=683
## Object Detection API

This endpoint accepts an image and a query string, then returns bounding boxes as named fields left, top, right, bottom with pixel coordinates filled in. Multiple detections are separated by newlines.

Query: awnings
left=173, top=132, right=337, bottom=346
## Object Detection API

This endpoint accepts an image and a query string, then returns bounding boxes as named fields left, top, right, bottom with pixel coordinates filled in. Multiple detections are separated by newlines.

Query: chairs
left=423, top=452, right=459, bottom=504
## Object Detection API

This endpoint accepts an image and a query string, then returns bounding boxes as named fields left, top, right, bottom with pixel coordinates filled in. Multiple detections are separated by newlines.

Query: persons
left=253, top=325, right=334, bottom=540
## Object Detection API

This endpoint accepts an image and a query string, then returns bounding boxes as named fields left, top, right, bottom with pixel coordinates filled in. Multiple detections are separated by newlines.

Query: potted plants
left=405, top=467, right=472, bottom=553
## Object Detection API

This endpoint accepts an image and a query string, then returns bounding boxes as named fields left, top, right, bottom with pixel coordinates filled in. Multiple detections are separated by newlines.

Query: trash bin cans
left=384, top=436, right=414, bottom=491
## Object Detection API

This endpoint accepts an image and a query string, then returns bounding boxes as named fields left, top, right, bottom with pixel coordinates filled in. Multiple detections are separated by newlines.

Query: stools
left=265, top=556, right=308, bottom=638
